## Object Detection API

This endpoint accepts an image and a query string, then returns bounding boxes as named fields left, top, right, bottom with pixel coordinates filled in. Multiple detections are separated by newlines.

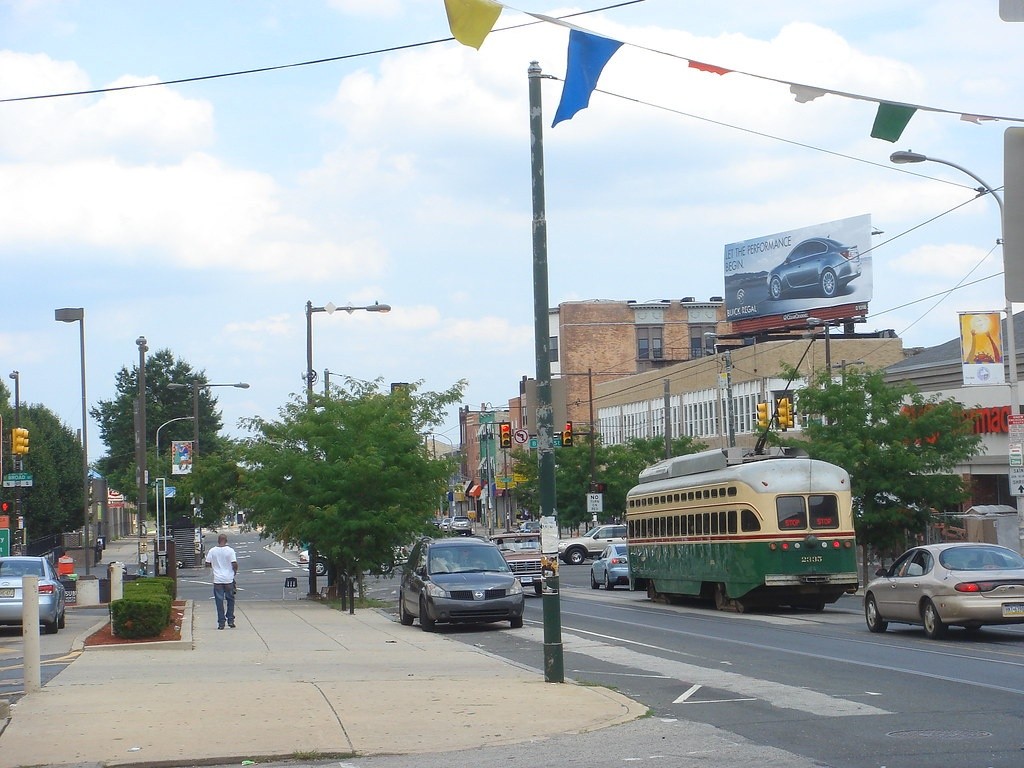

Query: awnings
left=446, top=481, right=512, bottom=501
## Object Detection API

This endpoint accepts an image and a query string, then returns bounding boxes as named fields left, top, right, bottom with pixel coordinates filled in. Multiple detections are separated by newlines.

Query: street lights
left=891, top=149, right=1023, bottom=565
left=420, top=432, right=457, bottom=515
left=8, top=370, right=23, bottom=557
left=303, top=301, right=391, bottom=603
left=831, top=358, right=865, bottom=438
left=54, top=306, right=90, bottom=575
left=805, top=317, right=833, bottom=426
left=156, top=416, right=194, bottom=478
left=166, top=381, right=252, bottom=568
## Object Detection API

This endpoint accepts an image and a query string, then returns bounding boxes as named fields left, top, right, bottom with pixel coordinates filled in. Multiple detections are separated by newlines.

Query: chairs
left=282, top=576, right=299, bottom=600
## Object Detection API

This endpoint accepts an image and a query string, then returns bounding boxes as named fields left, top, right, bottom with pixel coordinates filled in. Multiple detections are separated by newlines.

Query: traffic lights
left=561, top=420, right=573, bottom=446
left=500, top=421, right=512, bottom=449
left=2, top=500, right=13, bottom=513
left=776, top=398, right=794, bottom=429
left=12, top=428, right=30, bottom=454
left=757, top=401, right=769, bottom=429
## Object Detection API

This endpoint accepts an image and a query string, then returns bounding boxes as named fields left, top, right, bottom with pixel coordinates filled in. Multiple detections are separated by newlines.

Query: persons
left=205, top=534, right=238, bottom=629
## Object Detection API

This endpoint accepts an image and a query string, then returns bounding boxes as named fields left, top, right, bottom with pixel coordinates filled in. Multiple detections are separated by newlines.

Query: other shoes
left=218, top=625, right=225, bottom=629
left=228, top=621, right=235, bottom=627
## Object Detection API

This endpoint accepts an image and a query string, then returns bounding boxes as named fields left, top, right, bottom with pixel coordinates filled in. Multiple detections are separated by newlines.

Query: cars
left=398, top=536, right=525, bottom=633
left=590, top=544, right=628, bottom=591
left=0, top=555, right=67, bottom=635
left=425, top=515, right=472, bottom=536
left=765, top=237, right=862, bottom=299
left=513, top=522, right=540, bottom=542
left=863, top=542, right=1024, bottom=639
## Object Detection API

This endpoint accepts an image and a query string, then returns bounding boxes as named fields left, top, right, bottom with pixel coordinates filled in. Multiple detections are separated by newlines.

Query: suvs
left=558, top=524, right=628, bottom=565
left=488, top=533, right=542, bottom=596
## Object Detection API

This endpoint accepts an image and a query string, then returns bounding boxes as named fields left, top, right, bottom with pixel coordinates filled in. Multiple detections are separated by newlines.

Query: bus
left=625, top=449, right=859, bottom=612
